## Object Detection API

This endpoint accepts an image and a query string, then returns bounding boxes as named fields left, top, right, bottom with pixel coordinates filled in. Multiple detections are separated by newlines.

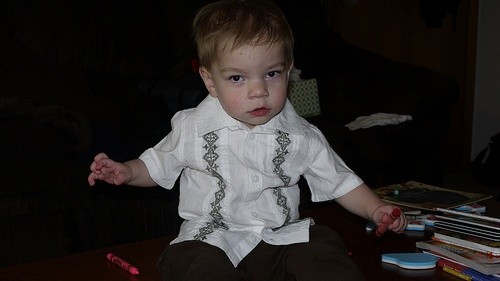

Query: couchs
left=1, top=0, right=459, bottom=268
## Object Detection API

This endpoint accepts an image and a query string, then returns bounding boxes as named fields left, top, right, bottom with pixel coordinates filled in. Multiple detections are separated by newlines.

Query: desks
left=0, top=198, right=500, bottom=281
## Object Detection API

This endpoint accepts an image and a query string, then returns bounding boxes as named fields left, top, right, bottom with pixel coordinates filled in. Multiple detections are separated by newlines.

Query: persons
left=88, top=0, right=408, bottom=281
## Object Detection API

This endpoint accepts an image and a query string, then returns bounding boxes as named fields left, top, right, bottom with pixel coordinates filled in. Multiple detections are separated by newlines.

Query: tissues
left=286, top=65, right=321, bottom=118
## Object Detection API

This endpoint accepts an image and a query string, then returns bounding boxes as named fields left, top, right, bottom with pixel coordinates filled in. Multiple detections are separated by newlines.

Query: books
left=416, top=206, right=500, bottom=281
